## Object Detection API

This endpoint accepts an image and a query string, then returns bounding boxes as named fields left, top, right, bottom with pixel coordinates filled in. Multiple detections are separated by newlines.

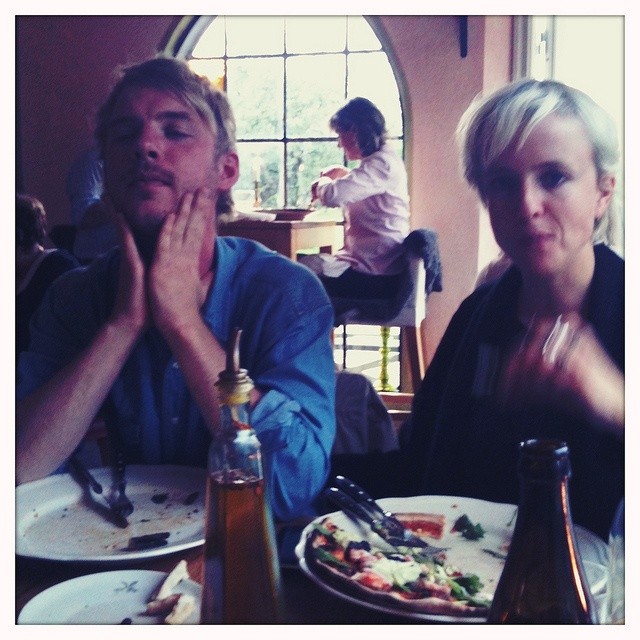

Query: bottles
left=196, top=331, right=282, bottom=625
left=485, top=437, right=598, bottom=625
left=604, top=498, right=625, bottom=624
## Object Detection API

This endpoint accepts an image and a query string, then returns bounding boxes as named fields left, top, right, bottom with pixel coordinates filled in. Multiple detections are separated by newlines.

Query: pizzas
left=303, top=515, right=494, bottom=614
left=393, top=510, right=448, bottom=539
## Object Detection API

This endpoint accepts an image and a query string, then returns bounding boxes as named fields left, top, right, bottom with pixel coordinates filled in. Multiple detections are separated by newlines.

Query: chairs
left=334, top=232, right=427, bottom=421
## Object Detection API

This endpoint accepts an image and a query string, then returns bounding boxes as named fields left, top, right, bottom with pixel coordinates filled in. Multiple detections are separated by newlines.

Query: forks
left=112, top=454, right=135, bottom=519
left=335, top=474, right=454, bottom=558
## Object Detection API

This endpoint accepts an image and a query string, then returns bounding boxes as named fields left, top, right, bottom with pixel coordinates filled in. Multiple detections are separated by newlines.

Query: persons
left=16, top=56, right=339, bottom=521
left=15, top=191, right=79, bottom=344
left=397, top=77, right=624, bottom=539
left=300, top=95, right=411, bottom=303
left=68, top=141, right=110, bottom=227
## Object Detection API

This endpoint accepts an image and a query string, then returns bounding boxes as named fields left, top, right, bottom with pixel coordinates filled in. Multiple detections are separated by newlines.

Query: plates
left=252, top=208, right=317, bottom=221
left=15, top=462, right=207, bottom=564
left=17, top=568, right=206, bottom=625
left=293, top=495, right=612, bottom=623
left=239, top=210, right=278, bottom=222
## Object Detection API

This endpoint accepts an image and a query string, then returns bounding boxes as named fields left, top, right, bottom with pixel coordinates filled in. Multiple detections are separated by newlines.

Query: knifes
left=324, top=486, right=424, bottom=559
left=70, top=456, right=129, bottom=527
